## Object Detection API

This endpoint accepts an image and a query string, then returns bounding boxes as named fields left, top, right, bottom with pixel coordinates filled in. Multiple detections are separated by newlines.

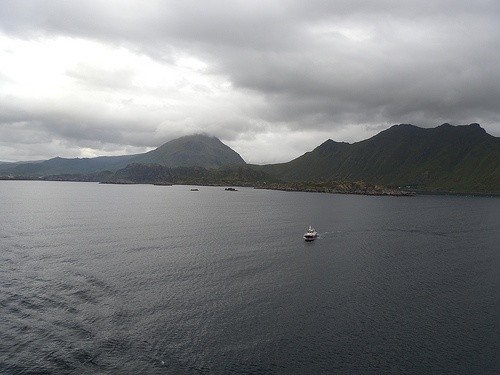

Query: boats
left=225, top=188, right=238, bottom=191
left=191, top=189, right=199, bottom=191
left=304, top=225, right=318, bottom=241
